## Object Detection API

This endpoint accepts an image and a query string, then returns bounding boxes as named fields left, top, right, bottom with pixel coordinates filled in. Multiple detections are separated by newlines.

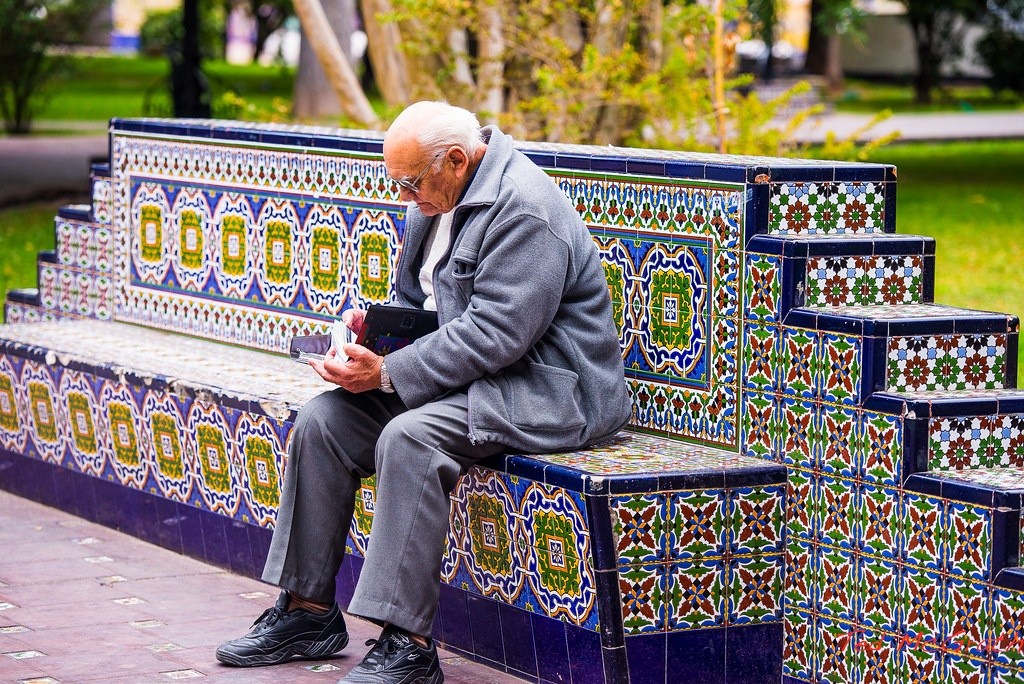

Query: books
left=333, top=305, right=440, bottom=362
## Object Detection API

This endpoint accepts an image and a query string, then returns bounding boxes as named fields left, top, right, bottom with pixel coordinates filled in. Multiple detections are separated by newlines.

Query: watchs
left=379, top=358, right=395, bottom=394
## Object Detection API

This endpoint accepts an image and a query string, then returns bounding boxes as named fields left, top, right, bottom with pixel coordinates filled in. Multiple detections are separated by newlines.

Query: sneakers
left=336, top=621, right=444, bottom=684
left=215, top=588, right=349, bottom=667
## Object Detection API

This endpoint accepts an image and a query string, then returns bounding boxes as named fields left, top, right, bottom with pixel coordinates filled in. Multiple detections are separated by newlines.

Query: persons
left=215, top=102, right=633, bottom=684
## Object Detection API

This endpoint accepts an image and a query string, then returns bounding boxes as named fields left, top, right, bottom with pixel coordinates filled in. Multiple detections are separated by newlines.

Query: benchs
left=0, top=115, right=1024, bottom=684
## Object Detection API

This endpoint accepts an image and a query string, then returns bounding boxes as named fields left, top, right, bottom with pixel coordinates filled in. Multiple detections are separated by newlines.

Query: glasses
left=391, top=148, right=449, bottom=195
